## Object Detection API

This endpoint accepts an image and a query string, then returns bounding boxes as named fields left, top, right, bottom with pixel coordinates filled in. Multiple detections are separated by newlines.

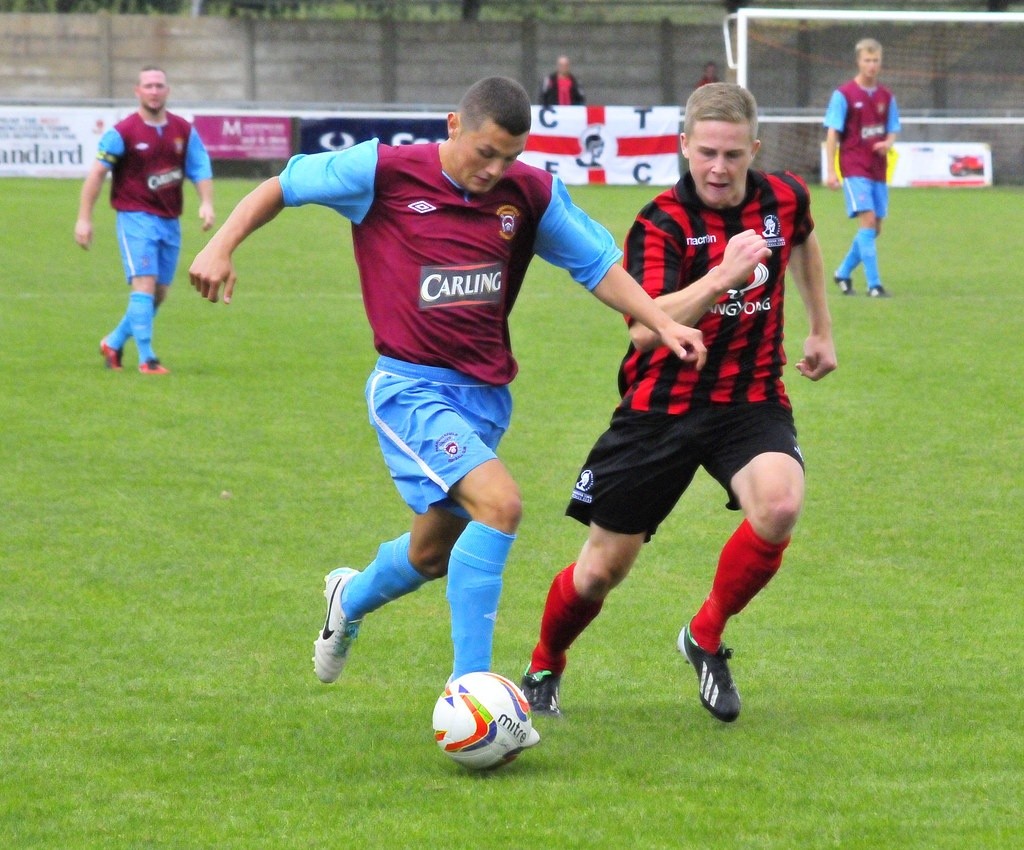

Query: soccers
left=431, top=670, right=534, bottom=771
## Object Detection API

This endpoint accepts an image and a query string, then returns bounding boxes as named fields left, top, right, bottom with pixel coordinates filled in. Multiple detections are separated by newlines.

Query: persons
left=75, top=65, right=214, bottom=375
left=823, top=39, right=900, bottom=299
left=521, top=84, right=837, bottom=724
left=190, top=78, right=707, bottom=749
left=537, top=54, right=587, bottom=106
left=696, top=61, right=718, bottom=87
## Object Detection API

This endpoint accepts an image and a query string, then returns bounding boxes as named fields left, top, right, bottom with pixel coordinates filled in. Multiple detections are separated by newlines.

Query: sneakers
left=520, top=662, right=562, bottom=719
left=866, top=285, right=891, bottom=297
left=311, top=567, right=362, bottom=683
left=140, top=359, right=169, bottom=374
left=99, top=337, right=122, bottom=370
left=833, top=270, right=855, bottom=295
left=677, top=614, right=741, bottom=722
left=445, top=673, right=540, bottom=747
